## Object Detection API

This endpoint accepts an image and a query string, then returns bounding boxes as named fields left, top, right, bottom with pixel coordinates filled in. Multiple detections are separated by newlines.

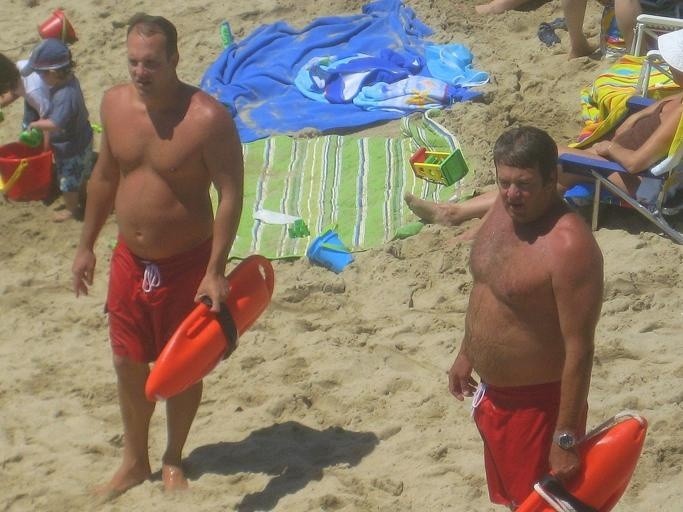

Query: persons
left=403, top=27, right=682, bottom=243
left=0, top=52, right=53, bottom=155
left=448, top=124, right=604, bottom=512
left=474, top=1, right=532, bottom=18
left=21, top=38, right=94, bottom=221
left=558, top=1, right=682, bottom=61
left=70, top=18, right=247, bottom=498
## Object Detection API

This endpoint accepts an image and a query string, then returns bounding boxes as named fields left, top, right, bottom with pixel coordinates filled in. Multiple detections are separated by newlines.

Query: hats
left=20, top=37, right=71, bottom=77
left=657, top=28, right=683, bottom=73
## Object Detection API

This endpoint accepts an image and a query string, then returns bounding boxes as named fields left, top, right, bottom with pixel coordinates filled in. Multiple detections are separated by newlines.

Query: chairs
left=557, top=14, right=683, bottom=245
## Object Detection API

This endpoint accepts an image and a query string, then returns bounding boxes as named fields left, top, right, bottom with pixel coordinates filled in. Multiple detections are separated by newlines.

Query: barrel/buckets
left=0, top=142, right=53, bottom=202
left=306, top=229, right=355, bottom=273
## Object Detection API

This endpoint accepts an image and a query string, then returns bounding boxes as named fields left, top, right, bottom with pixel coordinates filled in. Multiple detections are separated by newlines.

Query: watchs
left=551, top=430, right=578, bottom=454
